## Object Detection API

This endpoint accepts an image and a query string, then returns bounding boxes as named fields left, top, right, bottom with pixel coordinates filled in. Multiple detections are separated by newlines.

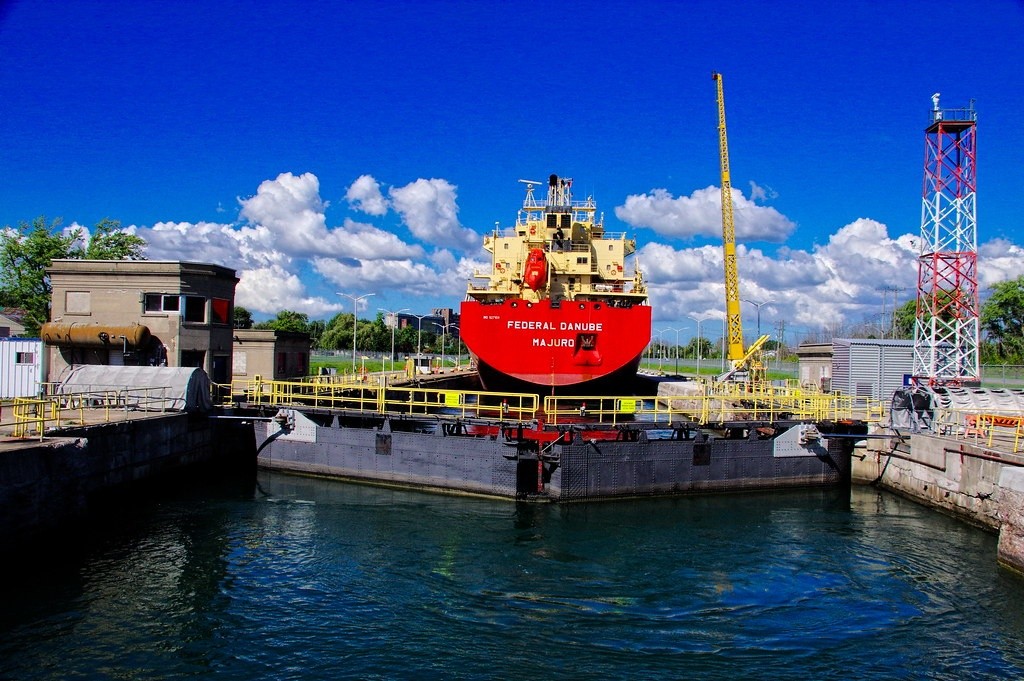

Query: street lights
left=688, top=316, right=714, bottom=380
left=450, top=326, right=460, bottom=371
left=740, top=299, right=775, bottom=341
left=377, top=308, right=410, bottom=373
left=668, top=327, right=690, bottom=376
left=432, top=322, right=456, bottom=372
left=716, top=315, right=728, bottom=374
left=336, top=292, right=375, bottom=374
left=653, top=328, right=672, bottom=375
left=406, top=313, right=434, bottom=374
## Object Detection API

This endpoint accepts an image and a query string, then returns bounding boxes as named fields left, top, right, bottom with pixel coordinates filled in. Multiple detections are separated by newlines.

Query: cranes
left=707, top=69, right=772, bottom=393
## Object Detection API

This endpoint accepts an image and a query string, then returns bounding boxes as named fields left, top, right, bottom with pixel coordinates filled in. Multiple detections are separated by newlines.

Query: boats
left=449, top=175, right=653, bottom=446
